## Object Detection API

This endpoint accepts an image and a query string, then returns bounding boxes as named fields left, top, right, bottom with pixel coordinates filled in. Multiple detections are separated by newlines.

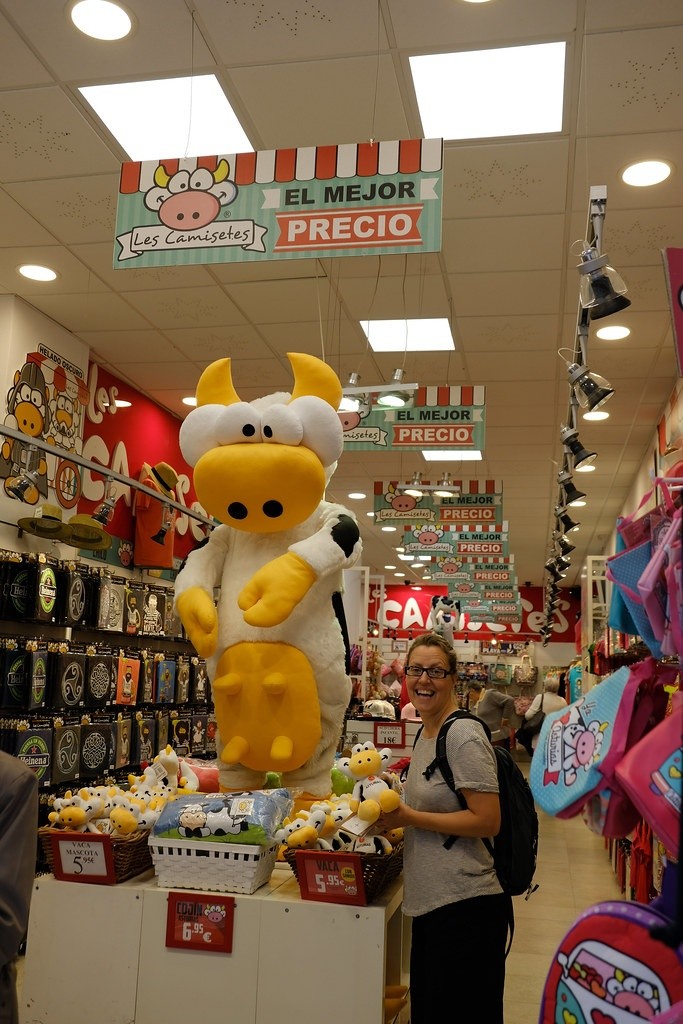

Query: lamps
left=91, top=496, right=115, bottom=526
left=525, top=636, right=529, bottom=645
left=540, top=625, right=548, bottom=635
left=555, top=554, right=571, bottom=572
left=387, top=627, right=391, bottom=637
left=549, top=602, right=559, bottom=611
left=544, top=564, right=566, bottom=583
left=377, top=368, right=408, bottom=406
left=6, top=469, right=40, bottom=502
left=557, top=470, right=586, bottom=505
left=150, top=521, right=171, bottom=545
left=550, top=594, right=560, bottom=604
left=550, top=582, right=562, bottom=595
left=392, top=629, right=397, bottom=640
left=555, top=505, right=580, bottom=535
left=408, top=631, right=413, bottom=640
left=434, top=472, right=454, bottom=497
left=404, top=471, right=424, bottom=496
left=553, top=530, right=576, bottom=556
left=579, top=240, right=631, bottom=321
left=464, top=633, right=469, bottom=643
left=565, top=360, right=615, bottom=412
left=338, top=372, right=363, bottom=412
left=559, top=421, right=598, bottom=469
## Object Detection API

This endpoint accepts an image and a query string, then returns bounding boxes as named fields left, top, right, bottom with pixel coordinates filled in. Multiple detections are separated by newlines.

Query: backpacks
left=412, top=711, right=539, bottom=897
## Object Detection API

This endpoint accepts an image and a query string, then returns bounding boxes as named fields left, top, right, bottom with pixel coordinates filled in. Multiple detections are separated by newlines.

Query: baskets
left=38, top=829, right=152, bottom=884
left=282, top=841, right=403, bottom=903
left=148, top=834, right=280, bottom=895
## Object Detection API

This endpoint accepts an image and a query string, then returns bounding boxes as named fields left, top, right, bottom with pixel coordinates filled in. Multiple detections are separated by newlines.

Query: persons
left=134, top=477, right=175, bottom=568
left=465, top=681, right=515, bottom=755
left=524, top=678, right=569, bottom=751
left=0, top=749, right=39, bottom=1024
left=375, top=634, right=508, bottom=1024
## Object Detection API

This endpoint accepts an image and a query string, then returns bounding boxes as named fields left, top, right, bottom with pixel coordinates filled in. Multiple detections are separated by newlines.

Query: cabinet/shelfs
left=0, top=461, right=683, bottom=1024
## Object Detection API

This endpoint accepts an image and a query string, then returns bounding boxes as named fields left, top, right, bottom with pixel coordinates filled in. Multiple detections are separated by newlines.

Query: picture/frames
left=391, top=640, right=408, bottom=652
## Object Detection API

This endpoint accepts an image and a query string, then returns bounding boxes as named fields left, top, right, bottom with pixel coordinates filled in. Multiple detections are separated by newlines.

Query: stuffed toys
left=275, top=742, right=405, bottom=855
left=47, top=745, right=200, bottom=837
left=169, top=352, right=360, bottom=817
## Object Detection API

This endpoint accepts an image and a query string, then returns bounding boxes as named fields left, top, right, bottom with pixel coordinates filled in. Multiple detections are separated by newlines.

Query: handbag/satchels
left=488, top=656, right=512, bottom=686
left=522, top=694, right=545, bottom=734
left=514, top=655, right=538, bottom=686
left=529, top=476, right=683, bottom=1023
left=514, top=686, right=535, bottom=716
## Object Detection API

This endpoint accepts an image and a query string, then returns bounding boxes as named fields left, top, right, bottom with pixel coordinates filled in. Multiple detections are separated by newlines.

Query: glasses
left=405, top=665, right=456, bottom=679
